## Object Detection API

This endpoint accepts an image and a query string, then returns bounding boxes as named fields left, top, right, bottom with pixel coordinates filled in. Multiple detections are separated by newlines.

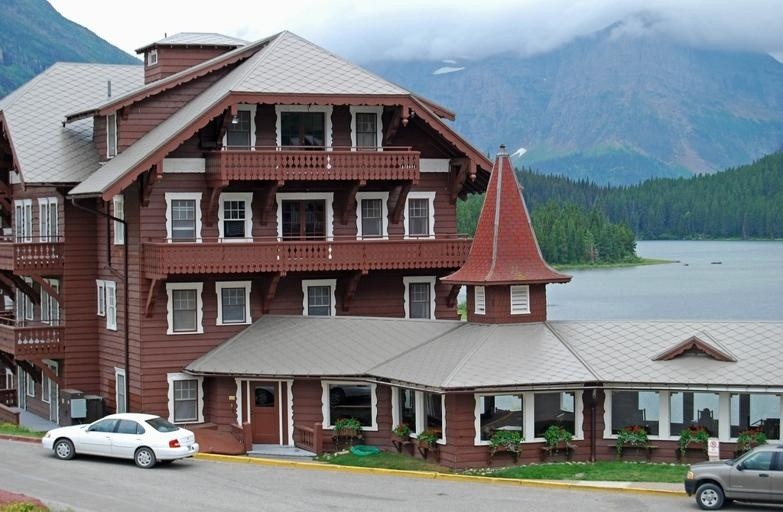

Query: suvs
left=684, top=443, right=783, bottom=510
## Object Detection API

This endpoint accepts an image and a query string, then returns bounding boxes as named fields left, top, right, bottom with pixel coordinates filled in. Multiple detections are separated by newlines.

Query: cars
left=39, top=411, right=200, bottom=470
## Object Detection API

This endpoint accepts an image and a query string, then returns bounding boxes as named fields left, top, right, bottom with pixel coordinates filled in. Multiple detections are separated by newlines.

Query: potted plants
left=333, top=417, right=363, bottom=440
left=391, top=424, right=438, bottom=450
left=489, top=425, right=769, bottom=459
